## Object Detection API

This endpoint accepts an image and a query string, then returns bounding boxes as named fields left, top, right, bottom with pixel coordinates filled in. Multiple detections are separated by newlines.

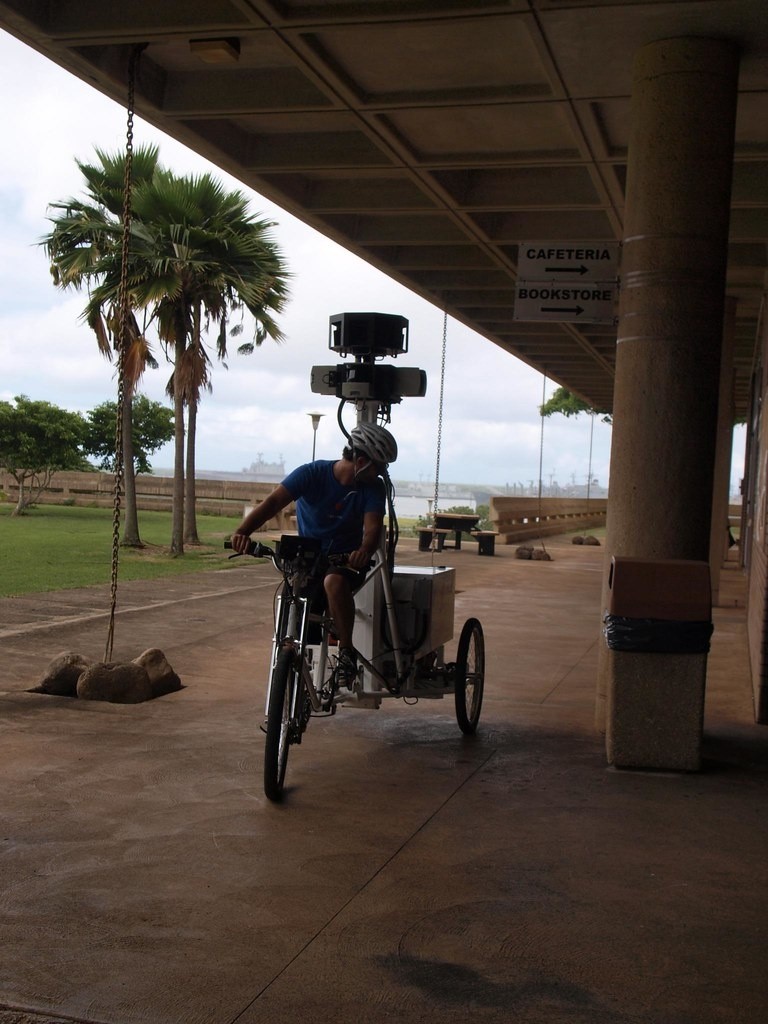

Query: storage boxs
left=381, top=565, right=456, bottom=658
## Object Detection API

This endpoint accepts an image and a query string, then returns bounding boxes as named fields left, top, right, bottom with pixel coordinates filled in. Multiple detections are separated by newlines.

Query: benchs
left=415, top=526, right=453, bottom=533
left=471, top=531, right=500, bottom=536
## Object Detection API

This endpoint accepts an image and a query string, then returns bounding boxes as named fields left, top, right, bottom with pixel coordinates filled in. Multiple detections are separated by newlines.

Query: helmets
left=347, top=420, right=397, bottom=464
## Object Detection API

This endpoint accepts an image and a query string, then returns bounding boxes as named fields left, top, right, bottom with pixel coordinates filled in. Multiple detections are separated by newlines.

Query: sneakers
left=335, top=647, right=357, bottom=687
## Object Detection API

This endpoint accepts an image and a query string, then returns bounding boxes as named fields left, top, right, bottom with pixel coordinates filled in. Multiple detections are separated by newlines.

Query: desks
left=419, top=513, right=495, bottom=556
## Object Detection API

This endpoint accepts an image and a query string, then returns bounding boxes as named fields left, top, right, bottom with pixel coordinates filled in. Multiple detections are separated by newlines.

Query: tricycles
left=223, top=541, right=484, bottom=800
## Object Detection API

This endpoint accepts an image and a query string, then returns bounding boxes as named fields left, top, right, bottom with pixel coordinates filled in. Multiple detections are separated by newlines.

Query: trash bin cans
left=601, top=554, right=715, bottom=773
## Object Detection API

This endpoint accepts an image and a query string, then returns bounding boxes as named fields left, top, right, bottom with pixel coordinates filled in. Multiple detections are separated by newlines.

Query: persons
left=232, top=422, right=398, bottom=687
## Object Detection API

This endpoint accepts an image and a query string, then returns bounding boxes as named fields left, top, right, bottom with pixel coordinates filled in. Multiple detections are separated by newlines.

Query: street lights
left=308, top=411, right=325, bottom=462
